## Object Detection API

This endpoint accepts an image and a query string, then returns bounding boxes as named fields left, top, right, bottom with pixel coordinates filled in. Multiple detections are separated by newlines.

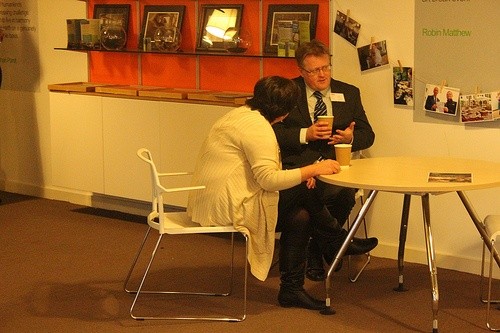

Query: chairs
left=267, top=150, right=370, bottom=282
left=480, top=215, right=500, bottom=331
left=123, top=148, right=248, bottom=322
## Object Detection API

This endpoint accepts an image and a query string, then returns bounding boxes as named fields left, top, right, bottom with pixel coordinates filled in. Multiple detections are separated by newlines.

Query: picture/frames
left=264, top=4, right=319, bottom=53
left=195, top=4, right=244, bottom=52
left=93, top=4, right=130, bottom=48
left=138, top=5, right=186, bottom=49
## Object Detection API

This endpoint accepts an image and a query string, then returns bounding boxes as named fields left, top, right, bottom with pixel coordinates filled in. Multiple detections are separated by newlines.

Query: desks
left=315, top=157, right=499, bottom=333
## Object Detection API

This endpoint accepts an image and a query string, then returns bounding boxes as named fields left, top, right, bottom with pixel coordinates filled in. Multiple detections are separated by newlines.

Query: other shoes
left=322, top=243, right=343, bottom=272
left=306, top=248, right=326, bottom=281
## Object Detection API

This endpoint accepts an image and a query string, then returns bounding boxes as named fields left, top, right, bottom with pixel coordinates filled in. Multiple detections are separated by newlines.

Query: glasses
left=299, top=64, right=331, bottom=74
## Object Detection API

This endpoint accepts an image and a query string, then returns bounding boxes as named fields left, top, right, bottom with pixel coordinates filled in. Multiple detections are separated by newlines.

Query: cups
left=317, top=116, right=334, bottom=137
left=334, top=144, right=352, bottom=168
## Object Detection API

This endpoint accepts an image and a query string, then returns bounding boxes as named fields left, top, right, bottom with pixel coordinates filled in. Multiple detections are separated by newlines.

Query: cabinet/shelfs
left=0, top=0, right=328, bottom=220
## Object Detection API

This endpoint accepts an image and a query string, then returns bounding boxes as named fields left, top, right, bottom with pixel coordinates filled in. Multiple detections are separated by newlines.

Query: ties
left=313, top=91, right=328, bottom=123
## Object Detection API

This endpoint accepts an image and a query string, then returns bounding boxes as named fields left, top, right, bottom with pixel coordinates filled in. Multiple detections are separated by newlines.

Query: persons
left=186, top=76, right=378, bottom=311
left=424, top=86, right=457, bottom=114
left=268, top=41, right=379, bottom=279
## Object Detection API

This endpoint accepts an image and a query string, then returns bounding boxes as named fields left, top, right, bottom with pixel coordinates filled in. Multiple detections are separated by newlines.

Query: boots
left=312, top=205, right=378, bottom=256
left=279, top=230, right=328, bottom=310
